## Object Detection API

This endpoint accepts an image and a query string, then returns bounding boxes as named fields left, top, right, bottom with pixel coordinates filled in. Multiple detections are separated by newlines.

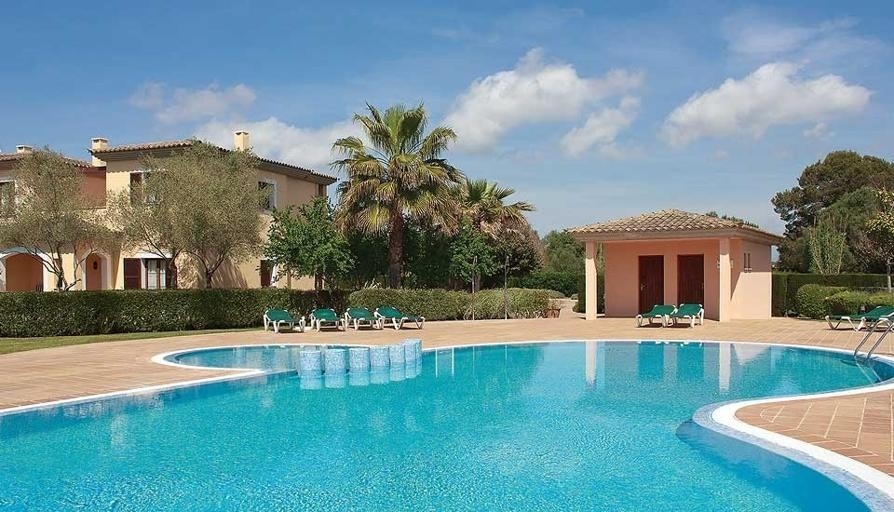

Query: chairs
left=635, top=301, right=704, bottom=332
left=826, top=306, right=894, bottom=334
left=262, top=303, right=426, bottom=331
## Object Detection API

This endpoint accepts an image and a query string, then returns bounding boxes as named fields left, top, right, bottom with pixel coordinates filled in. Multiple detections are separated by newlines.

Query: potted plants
left=545, top=290, right=563, bottom=319
